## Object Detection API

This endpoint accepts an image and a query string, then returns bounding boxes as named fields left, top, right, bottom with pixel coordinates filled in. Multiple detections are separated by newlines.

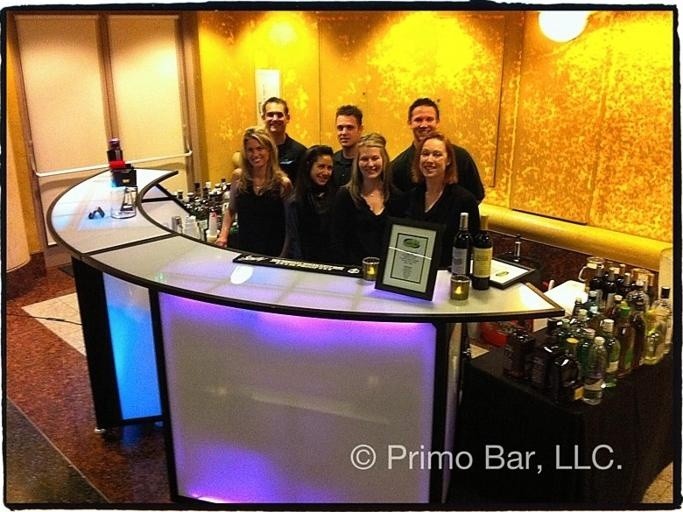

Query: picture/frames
left=374, top=216, right=447, bottom=301
left=448, top=254, right=536, bottom=289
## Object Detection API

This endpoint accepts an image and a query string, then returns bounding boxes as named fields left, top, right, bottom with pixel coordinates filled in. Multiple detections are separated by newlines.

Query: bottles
left=471, top=214, right=493, bottom=290
left=172, top=177, right=241, bottom=243
left=510, top=318, right=536, bottom=382
left=451, top=212, right=472, bottom=281
left=533, top=257, right=673, bottom=406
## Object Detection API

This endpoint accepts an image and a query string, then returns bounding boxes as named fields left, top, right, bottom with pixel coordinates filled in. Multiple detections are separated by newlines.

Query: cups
left=450, top=274, right=470, bottom=300
left=362, top=257, right=380, bottom=280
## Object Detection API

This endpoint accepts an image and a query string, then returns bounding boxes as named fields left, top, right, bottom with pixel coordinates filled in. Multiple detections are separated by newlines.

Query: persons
left=215, top=127, right=293, bottom=259
left=406, top=130, right=480, bottom=267
left=328, top=134, right=405, bottom=266
left=393, top=98, right=484, bottom=206
left=261, top=97, right=308, bottom=184
left=331, top=106, right=362, bottom=193
left=285, top=146, right=336, bottom=267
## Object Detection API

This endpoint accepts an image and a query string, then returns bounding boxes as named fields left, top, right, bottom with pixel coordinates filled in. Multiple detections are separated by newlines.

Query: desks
left=464, top=309, right=681, bottom=510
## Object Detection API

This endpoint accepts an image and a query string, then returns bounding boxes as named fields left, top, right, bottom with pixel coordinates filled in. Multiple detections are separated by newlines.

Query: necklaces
left=252, top=183, right=263, bottom=188
left=365, top=188, right=377, bottom=197
